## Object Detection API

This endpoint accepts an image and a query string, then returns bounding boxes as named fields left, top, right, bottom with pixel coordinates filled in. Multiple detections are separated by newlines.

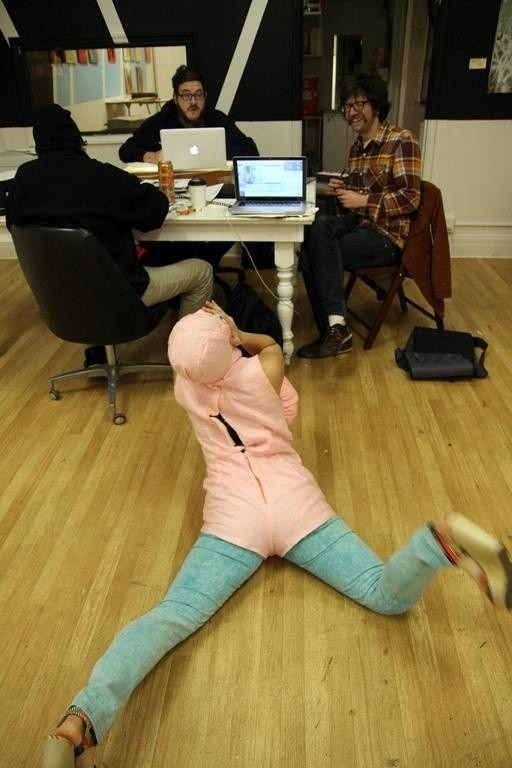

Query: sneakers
left=84, top=346, right=108, bottom=369
left=297, top=323, right=354, bottom=358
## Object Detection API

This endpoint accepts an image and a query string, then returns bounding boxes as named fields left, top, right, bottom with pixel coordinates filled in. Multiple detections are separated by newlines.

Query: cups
left=188, top=178, right=208, bottom=209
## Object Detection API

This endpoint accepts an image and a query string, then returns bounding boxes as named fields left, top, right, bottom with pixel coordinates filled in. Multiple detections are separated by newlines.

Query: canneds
left=158, top=160, right=174, bottom=205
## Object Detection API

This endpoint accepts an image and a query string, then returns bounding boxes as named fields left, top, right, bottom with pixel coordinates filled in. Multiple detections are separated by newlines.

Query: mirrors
left=9, top=33, right=199, bottom=136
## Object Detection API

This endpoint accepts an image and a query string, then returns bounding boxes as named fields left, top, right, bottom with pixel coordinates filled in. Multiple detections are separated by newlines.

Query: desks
left=135, top=177, right=318, bottom=367
left=104, top=99, right=168, bottom=119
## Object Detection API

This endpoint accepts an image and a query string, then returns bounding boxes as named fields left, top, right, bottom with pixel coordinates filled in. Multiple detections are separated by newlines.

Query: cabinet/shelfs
left=322, top=110, right=359, bottom=173
left=302, top=0, right=323, bottom=58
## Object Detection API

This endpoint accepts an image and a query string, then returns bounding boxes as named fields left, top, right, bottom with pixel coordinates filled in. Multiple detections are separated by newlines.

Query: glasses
left=340, top=100, right=369, bottom=112
left=177, top=89, right=208, bottom=100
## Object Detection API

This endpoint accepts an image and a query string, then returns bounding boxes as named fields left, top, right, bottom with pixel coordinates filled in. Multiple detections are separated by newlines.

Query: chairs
left=346, top=180, right=444, bottom=349
left=8, top=224, right=173, bottom=425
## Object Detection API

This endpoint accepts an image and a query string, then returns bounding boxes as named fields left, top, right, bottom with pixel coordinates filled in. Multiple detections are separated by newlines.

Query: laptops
left=160, top=127, right=227, bottom=172
left=229, top=156, right=306, bottom=214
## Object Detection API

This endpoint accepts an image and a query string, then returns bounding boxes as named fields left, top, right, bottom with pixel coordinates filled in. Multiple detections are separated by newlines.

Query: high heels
left=40, top=707, right=107, bottom=767
left=427, top=510, right=511, bottom=611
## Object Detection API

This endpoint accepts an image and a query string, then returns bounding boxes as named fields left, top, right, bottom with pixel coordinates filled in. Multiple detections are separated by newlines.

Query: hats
left=33, top=104, right=83, bottom=146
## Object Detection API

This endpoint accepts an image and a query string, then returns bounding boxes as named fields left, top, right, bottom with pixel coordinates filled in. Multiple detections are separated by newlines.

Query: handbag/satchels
left=394, top=325, right=489, bottom=380
left=225, top=282, right=283, bottom=358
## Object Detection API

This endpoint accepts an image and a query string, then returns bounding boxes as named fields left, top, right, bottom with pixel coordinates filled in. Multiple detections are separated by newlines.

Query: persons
left=6, top=102, right=215, bottom=318
left=297, top=72, right=421, bottom=358
left=39, top=299, right=512, bottom=768
left=118, top=65, right=260, bottom=264
